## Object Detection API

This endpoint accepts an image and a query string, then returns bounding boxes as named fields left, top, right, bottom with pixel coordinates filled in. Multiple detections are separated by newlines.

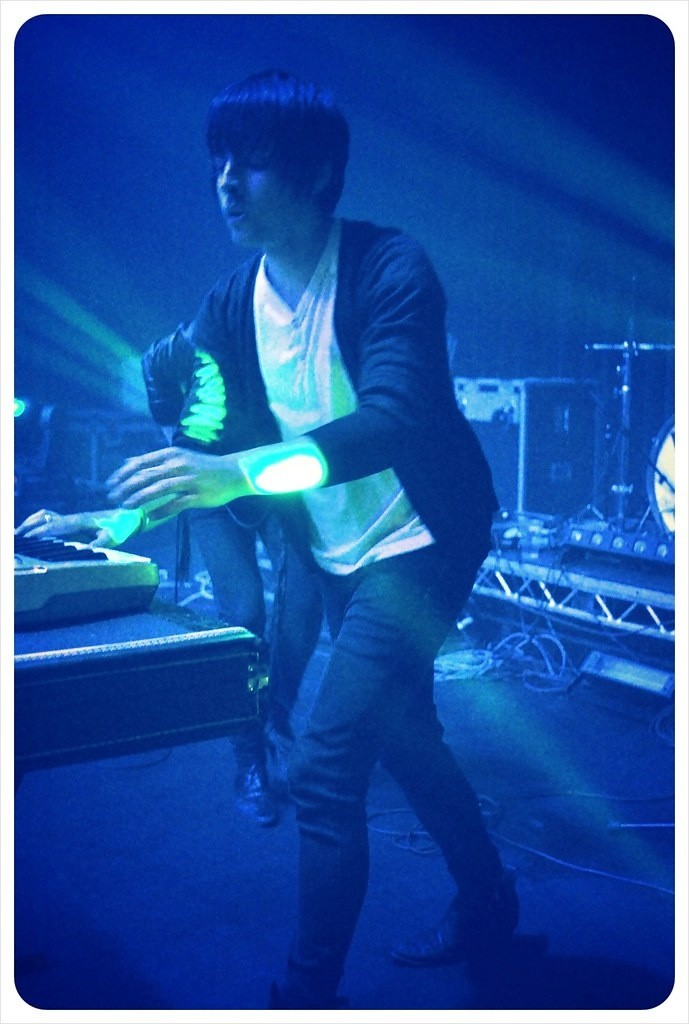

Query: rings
left=45, top=515, right=54, bottom=523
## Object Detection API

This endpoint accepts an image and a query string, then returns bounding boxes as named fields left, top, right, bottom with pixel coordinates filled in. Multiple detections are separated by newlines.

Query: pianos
left=13, top=529, right=159, bottom=633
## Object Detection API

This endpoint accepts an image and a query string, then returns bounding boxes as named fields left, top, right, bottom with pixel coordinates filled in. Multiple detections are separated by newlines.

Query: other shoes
left=233, top=764, right=277, bottom=824
left=265, top=976, right=336, bottom=1010
left=390, top=887, right=520, bottom=967
left=263, top=720, right=303, bottom=769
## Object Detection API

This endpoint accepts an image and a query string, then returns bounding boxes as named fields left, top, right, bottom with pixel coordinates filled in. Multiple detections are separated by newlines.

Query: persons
left=15, top=67, right=518, bottom=1010
left=142, top=323, right=321, bottom=827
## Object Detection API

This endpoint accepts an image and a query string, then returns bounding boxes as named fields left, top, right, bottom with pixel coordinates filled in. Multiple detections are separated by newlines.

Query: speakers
left=455, top=375, right=582, bottom=526
